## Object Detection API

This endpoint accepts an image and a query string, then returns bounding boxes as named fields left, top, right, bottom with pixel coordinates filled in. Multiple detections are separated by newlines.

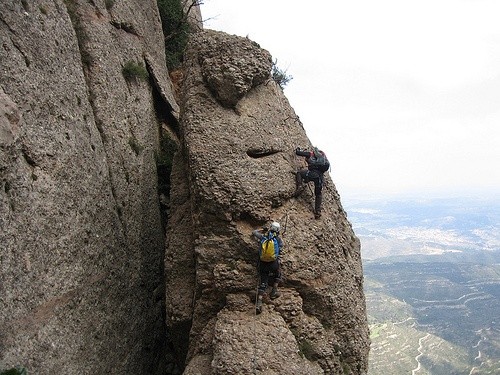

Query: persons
left=251, top=221, right=284, bottom=314
left=295, top=146, right=324, bottom=220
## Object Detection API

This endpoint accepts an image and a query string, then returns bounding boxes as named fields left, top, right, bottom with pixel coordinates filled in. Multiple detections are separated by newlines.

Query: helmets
left=271, top=222, right=280, bottom=231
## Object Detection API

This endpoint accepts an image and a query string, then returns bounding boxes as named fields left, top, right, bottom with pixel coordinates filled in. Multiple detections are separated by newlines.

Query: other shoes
left=270, top=291, right=281, bottom=299
left=256, top=306, right=262, bottom=313
left=315, top=211, right=321, bottom=217
left=297, top=185, right=303, bottom=191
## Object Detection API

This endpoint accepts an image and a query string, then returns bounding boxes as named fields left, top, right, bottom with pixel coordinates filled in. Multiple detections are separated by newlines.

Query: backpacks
left=259, top=234, right=278, bottom=262
left=310, top=149, right=329, bottom=172
